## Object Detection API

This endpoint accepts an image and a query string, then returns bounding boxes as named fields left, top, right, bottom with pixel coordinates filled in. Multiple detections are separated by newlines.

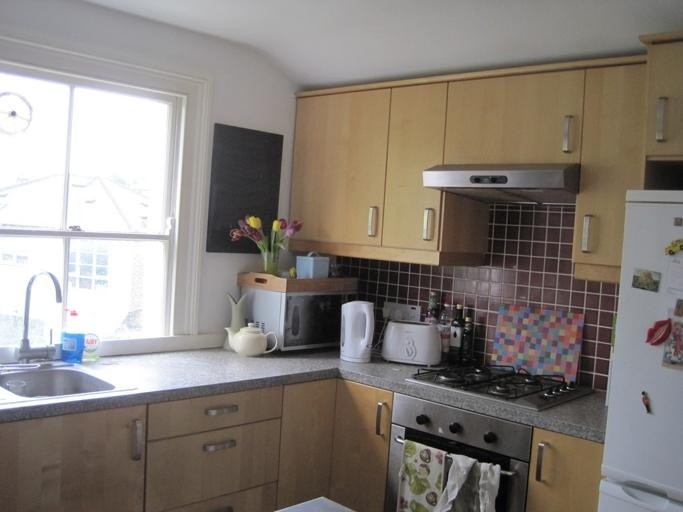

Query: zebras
left=384, top=424, right=533, bottom=511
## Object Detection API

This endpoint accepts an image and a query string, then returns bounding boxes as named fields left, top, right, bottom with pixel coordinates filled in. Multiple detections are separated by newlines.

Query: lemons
left=222, top=322, right=276, bottom=357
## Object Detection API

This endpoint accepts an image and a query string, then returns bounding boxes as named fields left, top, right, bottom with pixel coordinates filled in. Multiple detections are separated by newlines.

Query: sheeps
left=14, top=271, right=62, bottom=364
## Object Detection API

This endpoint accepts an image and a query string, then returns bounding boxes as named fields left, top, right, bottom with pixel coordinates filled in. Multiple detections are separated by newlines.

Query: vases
left=229, top=205, right=306, bottom=266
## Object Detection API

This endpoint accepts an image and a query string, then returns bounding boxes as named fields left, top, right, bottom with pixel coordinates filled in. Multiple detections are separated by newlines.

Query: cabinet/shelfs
left=425, top=289, right=472, bottom=360
left=62, top=310, right=83, bottom=362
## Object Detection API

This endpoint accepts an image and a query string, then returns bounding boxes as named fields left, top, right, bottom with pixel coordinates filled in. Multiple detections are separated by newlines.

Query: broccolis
left=594, top=188, right=683, bottom=512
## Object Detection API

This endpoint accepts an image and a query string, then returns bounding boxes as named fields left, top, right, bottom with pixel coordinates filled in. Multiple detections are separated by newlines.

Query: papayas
left=382, top=320, right=442, bottom=367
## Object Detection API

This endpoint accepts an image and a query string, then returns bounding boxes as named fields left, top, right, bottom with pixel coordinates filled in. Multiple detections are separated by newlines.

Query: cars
left=80, top=333, right=100, bottom=362
left=5, top=380, right=26, bottom=396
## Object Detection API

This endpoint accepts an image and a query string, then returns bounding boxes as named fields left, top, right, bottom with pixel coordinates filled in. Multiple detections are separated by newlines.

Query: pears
left=60, top=307, right=90, bottom=363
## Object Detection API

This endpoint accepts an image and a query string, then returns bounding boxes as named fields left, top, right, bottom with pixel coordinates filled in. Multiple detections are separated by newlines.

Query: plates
left=145, top=385, right=283, bottom=511
left=442, top=58, right=586, bottom=166
left=287, top=76, right=392, bottom=259
left=381, top=67, right=489, bottom=267
left=1, top=404, right=148, bottom=512
left=568, top=51, right=681, bottom=287
left=330, top=372, right=393, bottom=512
left=277, top=376, right=335, bottom=512
left=643, top=42, right=683, bottom=162
left=524, top=426, right=603, bottom=511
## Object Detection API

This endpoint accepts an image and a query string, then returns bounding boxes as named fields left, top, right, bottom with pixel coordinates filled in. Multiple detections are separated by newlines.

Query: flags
left=262, top=251, right=280, bottom=275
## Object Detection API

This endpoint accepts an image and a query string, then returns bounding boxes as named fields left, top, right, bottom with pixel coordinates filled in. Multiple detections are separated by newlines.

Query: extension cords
left=340, top=301, right=375, bottom=363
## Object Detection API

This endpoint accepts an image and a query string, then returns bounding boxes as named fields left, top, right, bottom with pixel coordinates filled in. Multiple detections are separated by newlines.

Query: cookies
left=236, top=286, right=358, bottom=352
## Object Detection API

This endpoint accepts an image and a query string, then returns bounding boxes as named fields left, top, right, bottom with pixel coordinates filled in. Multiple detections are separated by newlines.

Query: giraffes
left=422, top=164, right=579, bottom=204
left=390, top=357, right=594, bottom=464
